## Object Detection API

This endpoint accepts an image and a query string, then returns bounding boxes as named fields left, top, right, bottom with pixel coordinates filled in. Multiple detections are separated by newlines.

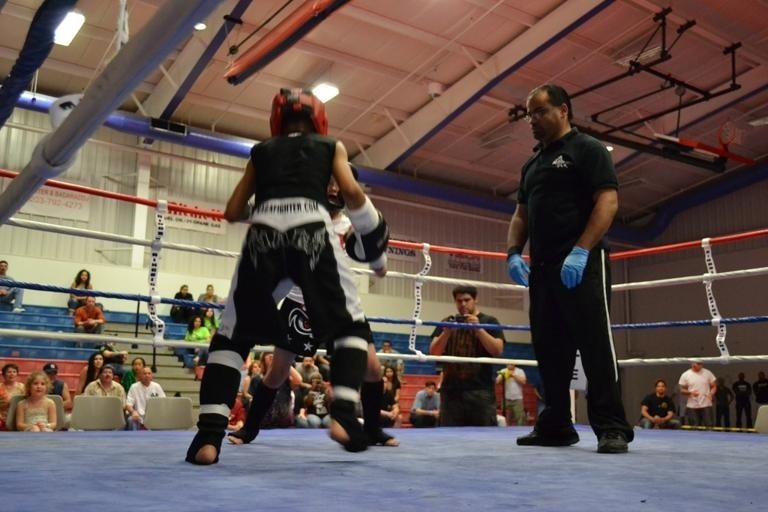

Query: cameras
left=316, top=381, right=321, bottom=384
left=456, top=316, right=466, bottom=322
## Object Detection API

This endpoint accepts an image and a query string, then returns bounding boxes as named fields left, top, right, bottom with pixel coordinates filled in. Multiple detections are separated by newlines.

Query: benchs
left=0, top=301, right=135, bottom=409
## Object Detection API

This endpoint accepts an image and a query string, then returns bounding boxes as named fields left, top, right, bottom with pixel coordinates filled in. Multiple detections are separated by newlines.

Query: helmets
left=325, top=162, right=359, bottom=210
left=267, top=86, right=328, bottom=139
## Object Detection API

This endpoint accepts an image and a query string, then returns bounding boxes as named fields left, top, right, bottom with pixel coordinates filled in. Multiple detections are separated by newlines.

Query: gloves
left=560, top=245, right=590, bottom=289
left=506, top=252, right=531, bottom=287
left=346, top=207, right=390, bottom=263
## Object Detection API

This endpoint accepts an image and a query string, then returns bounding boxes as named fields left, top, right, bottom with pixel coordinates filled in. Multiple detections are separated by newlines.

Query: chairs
left=5, top=394, right=194, bottom=430
left=755, top=405, right=768, bottom=434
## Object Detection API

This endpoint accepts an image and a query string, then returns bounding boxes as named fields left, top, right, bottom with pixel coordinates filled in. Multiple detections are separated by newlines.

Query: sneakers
left=517, top=423, right=581, bottom=447
left=596, top=430, right=629, bottom=454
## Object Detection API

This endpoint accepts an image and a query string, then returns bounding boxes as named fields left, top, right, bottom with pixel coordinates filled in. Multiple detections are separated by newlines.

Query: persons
left=183, top=86, right=389, bottom=465
left=228, top=154, right=402, bottom=447
left=375, top=283, right=528, bottom=427
left=504, top=83, right=635, bottom=456
left=637, top=361, right=763, bottom=433
left=176, top=284, right=331, bottom=429
left=0, top=259, right=170, bottom=431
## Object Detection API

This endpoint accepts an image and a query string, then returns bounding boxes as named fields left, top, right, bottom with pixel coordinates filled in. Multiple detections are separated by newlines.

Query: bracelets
left=506, top=244, right=523, bottom=258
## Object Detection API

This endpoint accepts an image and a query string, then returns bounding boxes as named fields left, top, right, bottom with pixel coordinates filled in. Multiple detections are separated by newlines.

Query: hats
left=43, top=363, right=58, bottom=374
